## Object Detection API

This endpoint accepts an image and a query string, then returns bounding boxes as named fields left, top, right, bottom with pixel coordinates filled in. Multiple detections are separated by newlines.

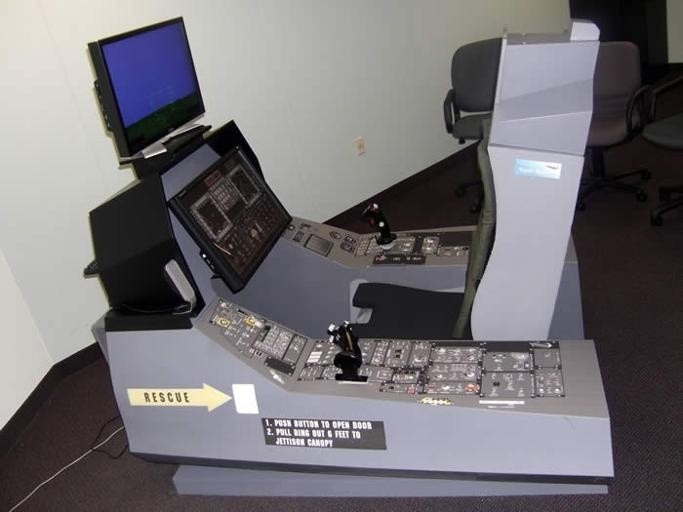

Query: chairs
left=576, top=41, right=683, bottom=227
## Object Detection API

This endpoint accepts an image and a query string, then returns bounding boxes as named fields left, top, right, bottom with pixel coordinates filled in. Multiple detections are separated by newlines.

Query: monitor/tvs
left=88, top=15, right=206, bottom=160
